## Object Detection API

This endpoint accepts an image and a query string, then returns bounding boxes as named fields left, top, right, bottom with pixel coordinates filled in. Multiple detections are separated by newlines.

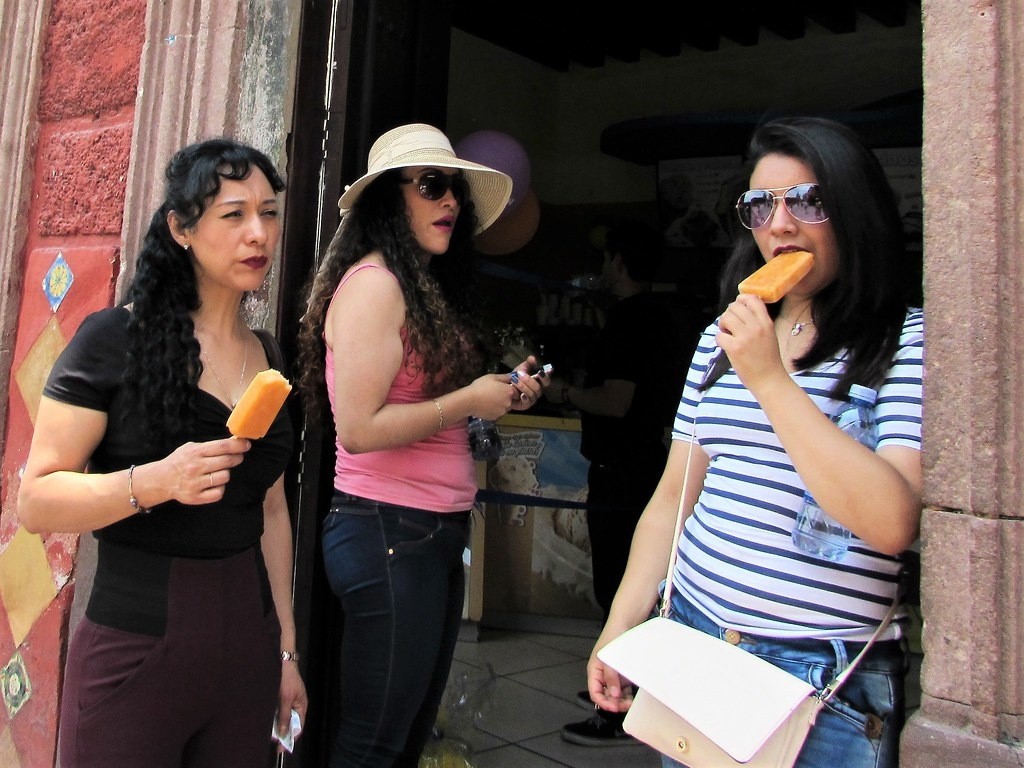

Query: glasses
left=735, top=183, right=833, bottom=231
left=397, top=168, right=466, bottom=205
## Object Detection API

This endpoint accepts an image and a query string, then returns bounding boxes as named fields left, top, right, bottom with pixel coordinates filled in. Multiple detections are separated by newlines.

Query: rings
left=209, top=472, right=214, bottom=487
left=604, top=686, right=607, bottom=688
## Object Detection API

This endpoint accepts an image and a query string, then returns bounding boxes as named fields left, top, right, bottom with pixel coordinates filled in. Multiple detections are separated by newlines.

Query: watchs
left=280, top=651, right=299, bottom=662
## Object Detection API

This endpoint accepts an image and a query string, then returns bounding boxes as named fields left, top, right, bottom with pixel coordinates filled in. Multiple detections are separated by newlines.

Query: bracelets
left=432, top=399, right=445, bottom=432
left=128, top=461, right=153, bottom=515
left=562, top=383, right=571, bottom=403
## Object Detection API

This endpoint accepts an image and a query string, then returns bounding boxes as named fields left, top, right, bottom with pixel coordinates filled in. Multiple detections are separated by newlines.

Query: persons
left=278, top=120, right=552, bottom=768
left=538, top=218, right=762, bottom=628
left=13, top=134, right=309, bottom=768
left=584, top=117, right=923, bottom=768
left=815, top=198, right=822, bottom=219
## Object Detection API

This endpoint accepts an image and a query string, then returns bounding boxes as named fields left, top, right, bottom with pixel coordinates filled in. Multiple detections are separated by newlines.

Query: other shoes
left=561, top=709, right=647, bottom=745
left=575, top=688, right=635, bottom=713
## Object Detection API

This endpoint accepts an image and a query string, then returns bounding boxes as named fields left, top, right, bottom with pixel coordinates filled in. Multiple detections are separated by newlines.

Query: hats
left=337, top=123, right=513, bottom=236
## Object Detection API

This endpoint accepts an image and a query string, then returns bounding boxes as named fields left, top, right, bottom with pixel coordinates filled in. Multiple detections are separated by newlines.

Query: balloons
left=454, top=129, right=539, bottom=255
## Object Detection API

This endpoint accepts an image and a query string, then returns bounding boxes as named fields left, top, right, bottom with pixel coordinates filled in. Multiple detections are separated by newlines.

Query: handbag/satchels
left=597, top=615, right=823, bottom=768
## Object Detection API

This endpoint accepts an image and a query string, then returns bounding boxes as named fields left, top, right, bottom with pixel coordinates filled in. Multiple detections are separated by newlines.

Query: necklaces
left=196, top=319, right=249, bottom=410
left=781, top=313, right=812, bottom=337
